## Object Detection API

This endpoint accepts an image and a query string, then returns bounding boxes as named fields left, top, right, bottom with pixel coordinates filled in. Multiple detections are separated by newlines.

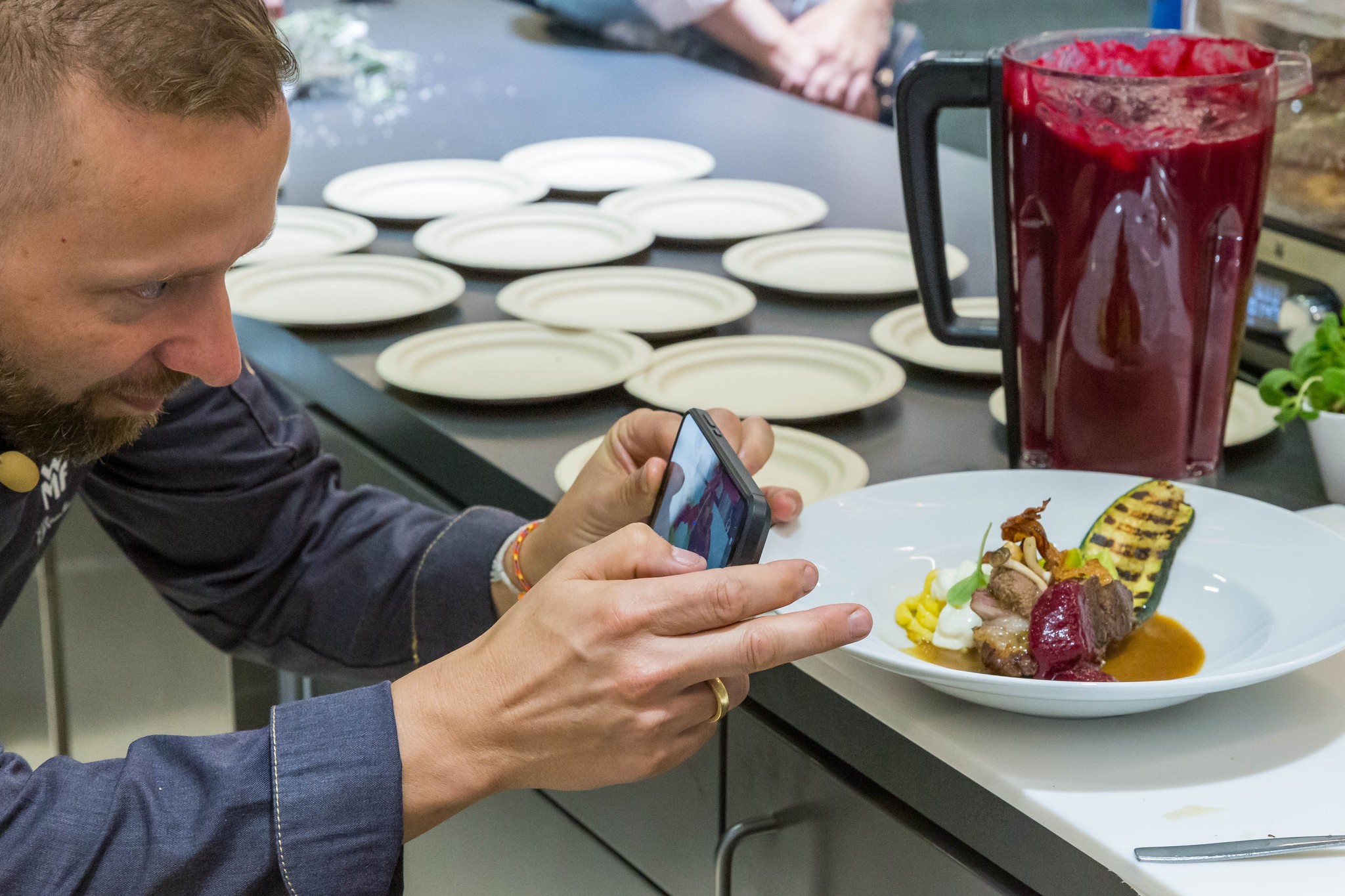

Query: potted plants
left=1259, top=307, right=1345, bottom=504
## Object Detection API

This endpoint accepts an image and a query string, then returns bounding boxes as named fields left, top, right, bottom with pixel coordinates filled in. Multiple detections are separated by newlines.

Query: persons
left=536, top=1, right=926, bottom=121
left=0, top=1, right=874, bottom=896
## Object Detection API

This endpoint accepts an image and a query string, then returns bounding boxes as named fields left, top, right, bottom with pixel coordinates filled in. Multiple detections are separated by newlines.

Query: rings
left=704, top=678, right=729, bottom=724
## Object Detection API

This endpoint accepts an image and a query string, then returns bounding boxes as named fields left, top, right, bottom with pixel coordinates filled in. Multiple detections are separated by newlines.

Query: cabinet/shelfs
left=304, top=406, right=1345, bottom=896
left=0, top=494, right=303, bottom=771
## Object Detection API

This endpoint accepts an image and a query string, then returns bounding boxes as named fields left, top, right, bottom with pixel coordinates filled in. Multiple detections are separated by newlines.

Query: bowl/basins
left=748, top=469, right=1344, bottom=720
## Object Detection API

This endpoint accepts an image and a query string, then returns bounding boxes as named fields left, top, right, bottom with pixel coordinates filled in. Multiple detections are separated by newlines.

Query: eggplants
left=1082, top=478, right=1195, bottom=626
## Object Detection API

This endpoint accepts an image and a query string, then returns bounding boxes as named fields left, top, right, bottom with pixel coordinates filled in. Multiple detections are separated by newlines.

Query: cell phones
left=647, top=407, right=772, bottom=569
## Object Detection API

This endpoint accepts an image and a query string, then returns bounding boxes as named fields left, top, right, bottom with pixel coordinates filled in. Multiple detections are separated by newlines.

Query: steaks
left=1025, top=576, right=1133, bottom=674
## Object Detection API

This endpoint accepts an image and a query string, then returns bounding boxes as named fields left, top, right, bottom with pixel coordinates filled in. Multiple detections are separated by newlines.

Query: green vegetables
left=945, top=522, right=993, bottom=609
left=1256, top=305, right=1344, bottom=430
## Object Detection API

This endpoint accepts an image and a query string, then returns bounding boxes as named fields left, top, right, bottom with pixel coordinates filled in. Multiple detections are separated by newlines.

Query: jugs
left=892, top=31, right=1316, bottom=484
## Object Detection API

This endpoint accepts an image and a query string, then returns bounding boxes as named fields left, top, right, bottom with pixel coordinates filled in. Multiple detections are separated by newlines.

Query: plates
left=223, top=136, right=1344, bottom=717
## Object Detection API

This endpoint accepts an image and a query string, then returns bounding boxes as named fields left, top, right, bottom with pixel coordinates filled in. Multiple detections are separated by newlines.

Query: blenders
left=1147, top=0, right=1344, bottom=403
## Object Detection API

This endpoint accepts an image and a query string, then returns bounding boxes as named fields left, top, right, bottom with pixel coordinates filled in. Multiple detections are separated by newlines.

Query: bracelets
left=490, top=518, right=545, bottom=599
